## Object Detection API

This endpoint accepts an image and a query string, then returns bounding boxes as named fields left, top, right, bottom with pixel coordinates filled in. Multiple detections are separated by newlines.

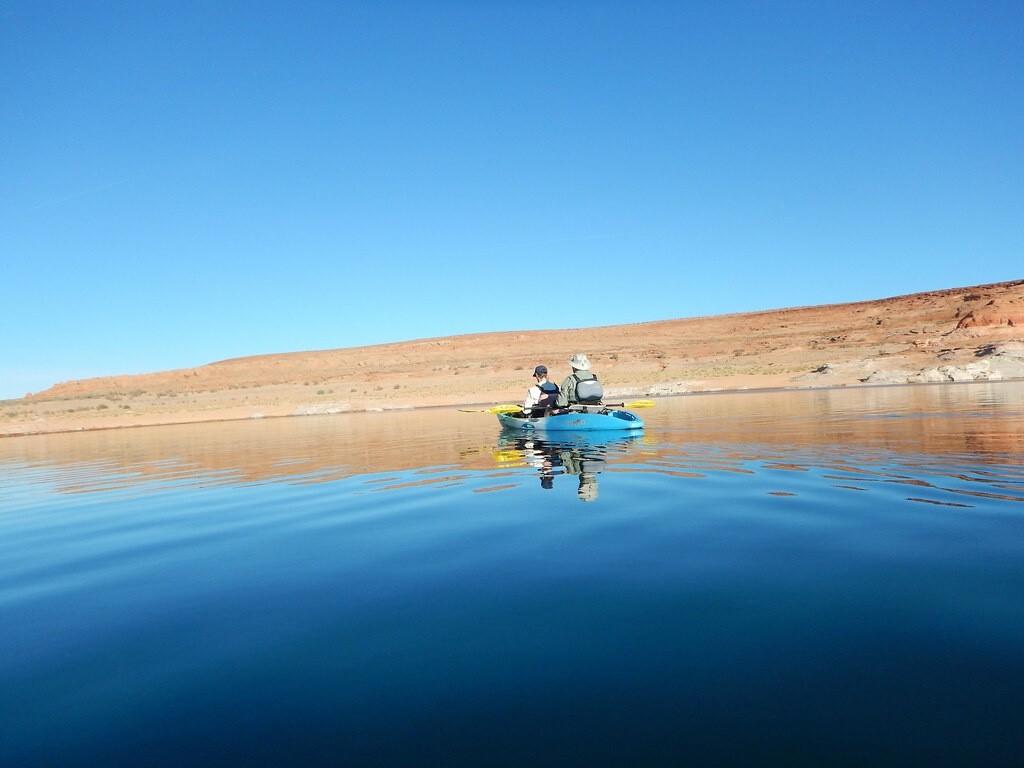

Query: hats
left=567, top=354, right=591, bottom=370
left=533, top=365, right=547, bottom=377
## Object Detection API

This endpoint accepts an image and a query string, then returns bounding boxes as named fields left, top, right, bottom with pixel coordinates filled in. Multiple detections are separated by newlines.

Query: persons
left=552, top=354, right=602, bottom=416
left=522, top=365, right=558, bottom=418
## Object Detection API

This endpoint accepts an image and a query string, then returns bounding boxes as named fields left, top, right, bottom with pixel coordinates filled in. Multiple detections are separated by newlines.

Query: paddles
left=488, top=399, right=656, bottom=414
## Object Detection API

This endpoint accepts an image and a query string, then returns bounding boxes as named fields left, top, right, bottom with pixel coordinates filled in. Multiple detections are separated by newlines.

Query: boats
left=500, top=431, right=646, bottom=458
left=497, top=407, right=645, bottom=431
